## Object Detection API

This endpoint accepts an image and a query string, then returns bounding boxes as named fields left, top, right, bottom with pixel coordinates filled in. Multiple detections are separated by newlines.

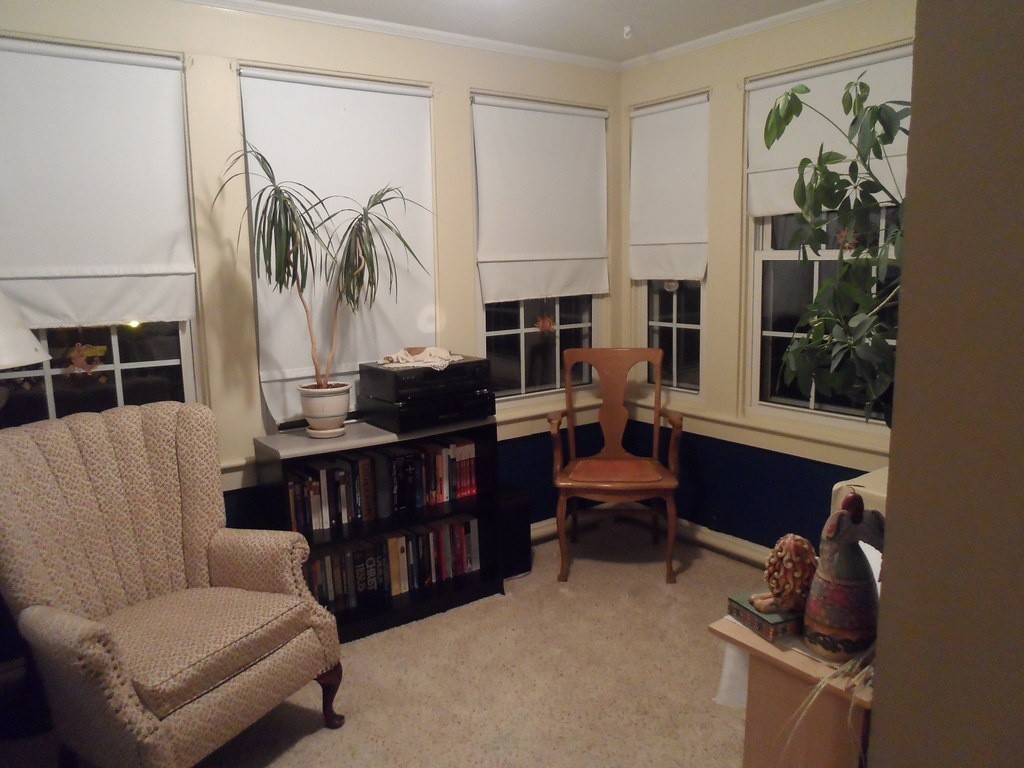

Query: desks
left=706, top=616, right=872, bottom=768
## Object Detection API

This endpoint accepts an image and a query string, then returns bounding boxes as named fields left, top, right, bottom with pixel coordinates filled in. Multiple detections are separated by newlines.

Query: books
left=287, top=439, right=477, bottom=531
left=303, top=514, right=481, bottom=612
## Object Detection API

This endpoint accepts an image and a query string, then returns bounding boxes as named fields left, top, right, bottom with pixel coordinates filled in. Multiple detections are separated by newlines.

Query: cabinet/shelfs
left=252, top=416, right=504, bottom=643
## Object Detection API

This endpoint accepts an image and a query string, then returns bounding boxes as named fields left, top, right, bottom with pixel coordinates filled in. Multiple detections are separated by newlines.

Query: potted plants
left=209, top=129, right=436, bottom=441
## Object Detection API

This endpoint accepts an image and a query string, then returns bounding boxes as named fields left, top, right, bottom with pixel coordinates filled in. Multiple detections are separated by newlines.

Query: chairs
left=550, top=346, right=687, bottom=586
left=0, top=402, right=346, bottom=768
